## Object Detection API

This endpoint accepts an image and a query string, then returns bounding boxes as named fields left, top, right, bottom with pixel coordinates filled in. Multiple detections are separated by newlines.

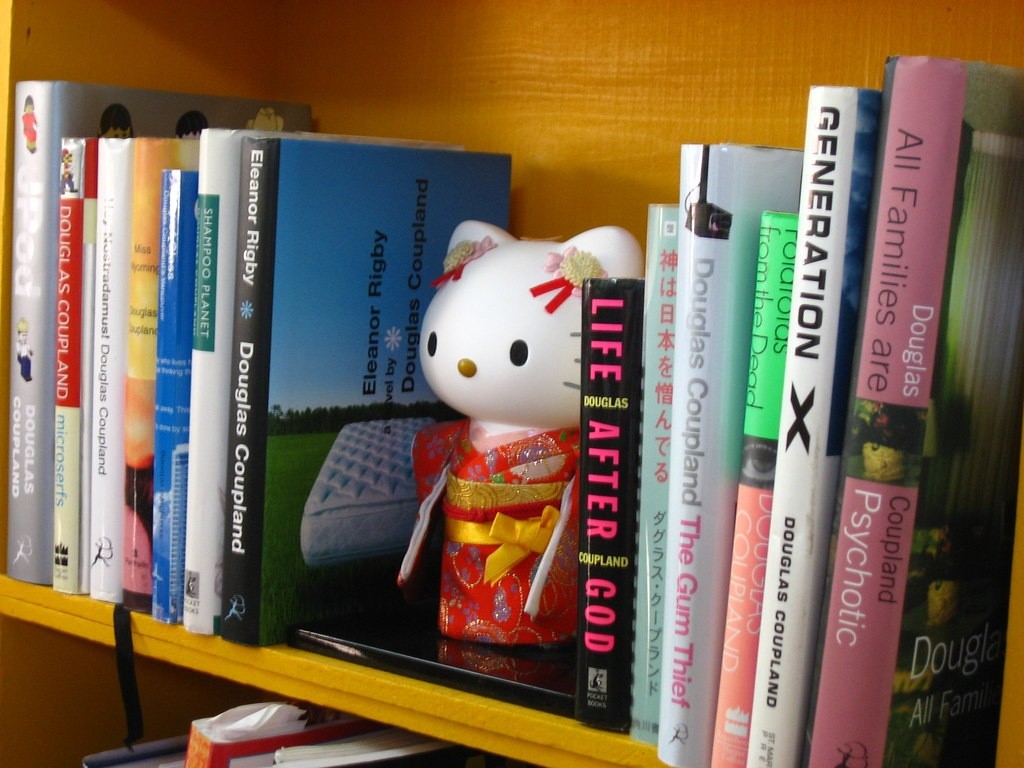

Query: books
left=8, top=55, right=1023, bottom=768
